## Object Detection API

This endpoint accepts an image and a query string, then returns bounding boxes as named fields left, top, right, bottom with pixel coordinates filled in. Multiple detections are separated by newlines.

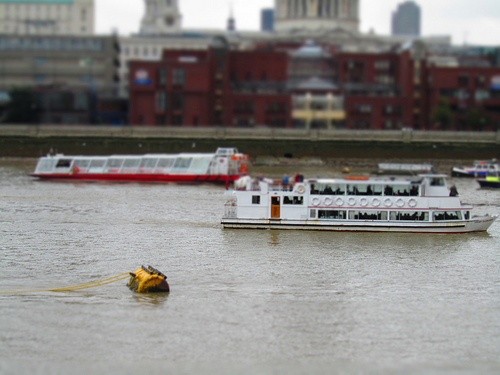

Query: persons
left=281, top=174, right=304, bottom=192
left=309, top=178, right=469, bottom=220
left=236, top=174, right=263, bottom=191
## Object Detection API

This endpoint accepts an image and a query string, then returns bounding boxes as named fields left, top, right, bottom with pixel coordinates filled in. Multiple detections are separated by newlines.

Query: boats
left=478, top=174, right=499, bottom=187
left=450, top=161, right=499, bottom=175
left=378, top=162, right=432, bottom=175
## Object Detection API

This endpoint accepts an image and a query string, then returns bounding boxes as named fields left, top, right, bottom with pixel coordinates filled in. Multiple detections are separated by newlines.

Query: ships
left=219, top=164, right=496, bottom=234
left=29, top=148, right=246, bottom=184
